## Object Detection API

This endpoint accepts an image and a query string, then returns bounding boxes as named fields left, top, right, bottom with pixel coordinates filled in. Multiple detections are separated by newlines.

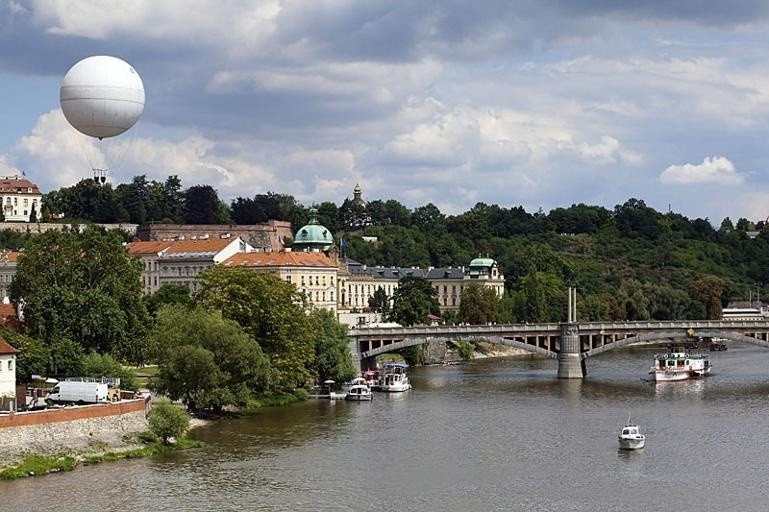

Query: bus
left=64, top=376, right=122, bottom=401
left=720, top=307, right=765, bottom=321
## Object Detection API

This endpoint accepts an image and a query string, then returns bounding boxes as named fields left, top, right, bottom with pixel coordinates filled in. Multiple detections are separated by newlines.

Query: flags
left=341, top=238, right=349, bottom=247
left=278, top=236, right=286, bottom=245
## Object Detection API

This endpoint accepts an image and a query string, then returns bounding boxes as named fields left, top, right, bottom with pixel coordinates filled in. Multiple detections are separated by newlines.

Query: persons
left=112, top=394, right=117, bottom=402
left=132, top=392, right=144, bottom=399
left=106, top=392, right=110, bottom=401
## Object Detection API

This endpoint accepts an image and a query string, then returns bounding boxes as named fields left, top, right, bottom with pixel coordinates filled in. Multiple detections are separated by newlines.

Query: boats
left=666, top=341, right=700, bottom=349
left=618, top=411, right=648, bottom=450
left=347, top=363, right=412, bottom=402
left=709, top=343, right=729, bottom=351
left=640, top=345, right=712, bottom=385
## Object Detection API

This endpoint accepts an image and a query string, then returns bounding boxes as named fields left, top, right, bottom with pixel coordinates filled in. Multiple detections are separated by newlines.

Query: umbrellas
left=324, top=379, right=335, bottom=394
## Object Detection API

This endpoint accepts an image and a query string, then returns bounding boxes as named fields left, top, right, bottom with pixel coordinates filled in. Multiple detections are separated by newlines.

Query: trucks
left=43, top=380, right=109, bottom=407
